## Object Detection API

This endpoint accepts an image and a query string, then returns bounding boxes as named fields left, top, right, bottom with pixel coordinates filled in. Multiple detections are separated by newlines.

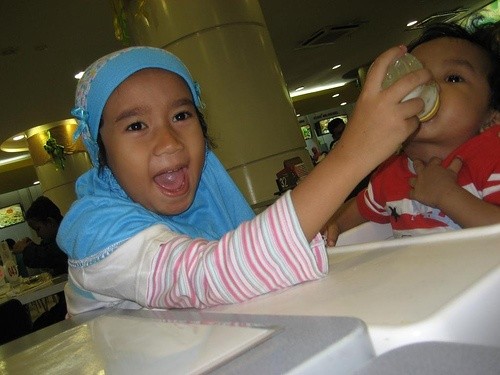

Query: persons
left=13, top=196, right=69, bottom=277
left=318, top=22, right=500, bottom=248
left=327, top=118, right=346, bottom=149
left=56, top=44, right=432, bottom=320
left=6, top=238, right=28, bottom=278
left=310, top=147, right=320, bottom=161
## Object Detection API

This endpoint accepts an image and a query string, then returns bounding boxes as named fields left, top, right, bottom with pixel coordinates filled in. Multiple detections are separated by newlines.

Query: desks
left=0, top=308, right=499, bottom=375
left=250, top=194, right=281, bottom=209
left=0, top=274, right=68, bottom=305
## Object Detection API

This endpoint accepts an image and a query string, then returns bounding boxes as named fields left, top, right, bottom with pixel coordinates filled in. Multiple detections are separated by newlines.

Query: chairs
left=200, top=221, right=500, bottom=355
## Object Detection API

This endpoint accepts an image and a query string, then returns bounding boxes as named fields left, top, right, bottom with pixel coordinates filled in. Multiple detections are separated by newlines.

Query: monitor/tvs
left=319, top=114, right=348, bottom=135
left=0, top=203, right=26, bottom=229
left=301, top=124, right=312, bottom=140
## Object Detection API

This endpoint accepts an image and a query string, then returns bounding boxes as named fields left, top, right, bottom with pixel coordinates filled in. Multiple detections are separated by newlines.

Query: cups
left=367, top=53, right=440, bottom=122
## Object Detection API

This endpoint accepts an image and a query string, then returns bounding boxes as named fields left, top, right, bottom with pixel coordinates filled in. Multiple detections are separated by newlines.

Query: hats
left=69, top=45, right=207, bottom=195
